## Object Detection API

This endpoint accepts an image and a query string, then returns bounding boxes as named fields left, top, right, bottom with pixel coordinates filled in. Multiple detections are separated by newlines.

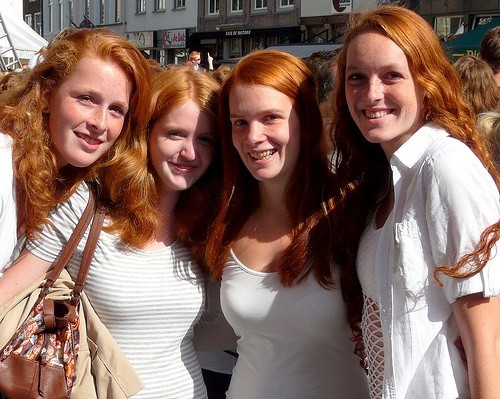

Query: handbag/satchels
left=1, top=181, right=105, bottom=399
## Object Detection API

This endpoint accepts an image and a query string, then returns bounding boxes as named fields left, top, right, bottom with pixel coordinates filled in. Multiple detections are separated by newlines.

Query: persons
left=0, top=26, right=158, bottom=281
left=184, top=24, right=500, bottom=177
left=326, top=6, right=500, bottom=399
left=1, top=64, right=371, bottom=399
left=189, top=48, right=371, bottom=399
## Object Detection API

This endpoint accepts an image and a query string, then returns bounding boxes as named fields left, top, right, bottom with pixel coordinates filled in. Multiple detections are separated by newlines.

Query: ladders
left=0, top=15, right=23, bottom=71
